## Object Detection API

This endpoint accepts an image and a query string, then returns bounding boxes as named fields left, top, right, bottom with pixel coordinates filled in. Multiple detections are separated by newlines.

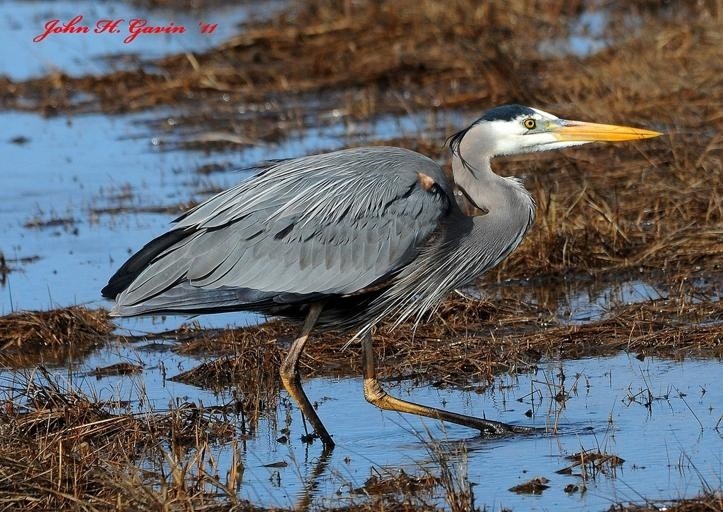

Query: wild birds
left=101, top=103, right=664, bottom=445
